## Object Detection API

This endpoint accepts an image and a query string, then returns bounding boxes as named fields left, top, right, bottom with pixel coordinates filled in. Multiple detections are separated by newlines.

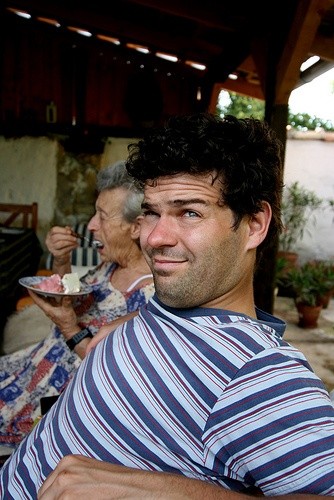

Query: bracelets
left=66, top=329, right=92, bottom=351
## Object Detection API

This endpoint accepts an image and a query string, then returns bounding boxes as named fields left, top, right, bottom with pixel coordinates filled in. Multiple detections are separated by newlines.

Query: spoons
left=71, top=231, right=103, bottom=246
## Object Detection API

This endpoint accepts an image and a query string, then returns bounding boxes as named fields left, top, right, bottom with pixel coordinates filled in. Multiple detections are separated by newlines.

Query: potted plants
left=275, top=181, right=334, bottom=271
left=286, top=267, right=323, bottom=327
left=301, top=261, right=334, bottom=309
left=277, top=268, right=302, bottom=297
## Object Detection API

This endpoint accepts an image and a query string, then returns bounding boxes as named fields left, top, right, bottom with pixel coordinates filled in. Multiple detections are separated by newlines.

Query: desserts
left=38, top=273, right=81, bottom=293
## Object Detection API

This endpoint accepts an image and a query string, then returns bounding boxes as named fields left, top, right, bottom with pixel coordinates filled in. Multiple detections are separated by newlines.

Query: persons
left=0, top=113, right=334, bottom=500
left=0, top=158, right=156, bottom=445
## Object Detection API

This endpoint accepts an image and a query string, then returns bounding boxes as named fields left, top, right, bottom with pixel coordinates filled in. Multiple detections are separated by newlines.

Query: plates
left=19, top=276, right=93, bottom=298
